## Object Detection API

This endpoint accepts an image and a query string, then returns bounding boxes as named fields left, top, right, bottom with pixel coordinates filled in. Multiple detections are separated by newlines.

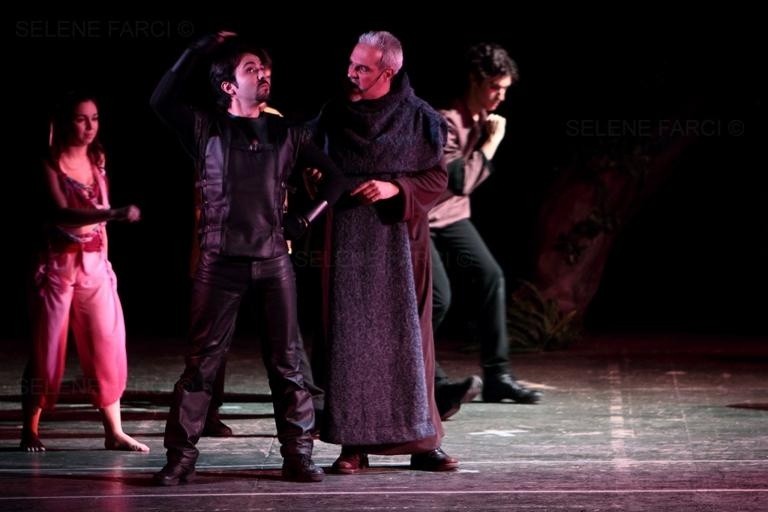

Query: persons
left=151, top=30, right=327, bottom=487
left=429, top=42, right=544, bottom=420
left=298, top=91, right=485, bottom=422
left=289, top=32, right=462, bottom=472
left=19, top=95, right=151, bottom=452
left=198, top=45, right=329, bottom=440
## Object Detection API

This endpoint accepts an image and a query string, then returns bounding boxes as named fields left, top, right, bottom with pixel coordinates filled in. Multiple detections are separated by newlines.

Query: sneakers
left=151, top=461, right=196, bottom=486
left=203, top=420, right=233, bottom=438
left=436, top=374, right=483, bottom=420
left=410, top=447, right=461, bottom=472
left=331, top=446, right=369, bottom=475
left=281, top=454, right=325, bottom=483
left=483, top=372, right=544, bottom=403
